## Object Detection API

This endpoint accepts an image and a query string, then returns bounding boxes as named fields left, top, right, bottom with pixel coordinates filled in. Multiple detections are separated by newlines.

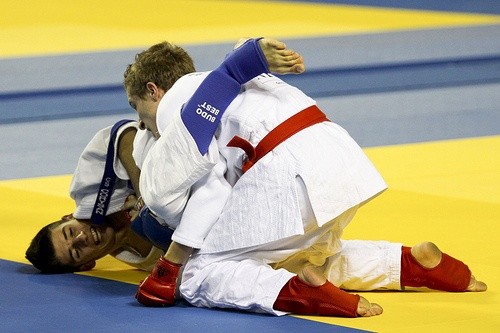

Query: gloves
left=129, top=195, right=174, bottom=254
left=135, top=256, right=183, bottom=306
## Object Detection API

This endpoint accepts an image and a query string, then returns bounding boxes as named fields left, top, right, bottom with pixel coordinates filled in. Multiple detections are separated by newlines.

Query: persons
left=123, top=40, right=487, bottom=316
left=25, top=37, right=304, bottom=272
